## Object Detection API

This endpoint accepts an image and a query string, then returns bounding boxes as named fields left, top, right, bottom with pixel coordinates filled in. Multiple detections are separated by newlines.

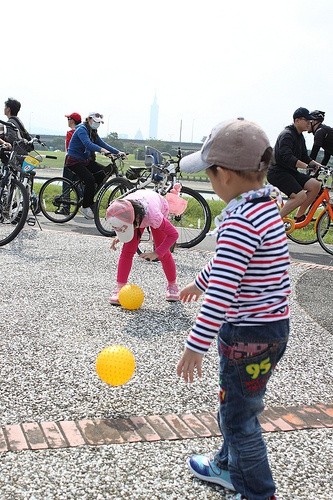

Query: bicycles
left=0, top=120, right=56, bottom=246
left=94, top=146, right=210, bottom=248
left=39, top=152, right=135, bottom=223
left=270, top=164, right=333, bottom=255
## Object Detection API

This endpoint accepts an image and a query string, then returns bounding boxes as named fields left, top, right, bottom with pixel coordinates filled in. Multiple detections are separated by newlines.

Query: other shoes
left=33, top=197, right=42, bottom=214
left=57, top=208, right=71, bottom=214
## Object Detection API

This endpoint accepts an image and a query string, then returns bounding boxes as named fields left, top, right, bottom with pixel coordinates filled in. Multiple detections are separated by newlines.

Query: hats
left=180, top=117, right=273, bottom=174
left=293, top=107, right=315, bottom=121
left=106, top=199, right=134, bottom=243
left=65, top=112, right=81, bottom=121
left=88, top=112, right=104, bottom=124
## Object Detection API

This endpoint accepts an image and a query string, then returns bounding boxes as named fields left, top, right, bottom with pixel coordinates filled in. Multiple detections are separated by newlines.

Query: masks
left=89, top=121, right=100, bottom=129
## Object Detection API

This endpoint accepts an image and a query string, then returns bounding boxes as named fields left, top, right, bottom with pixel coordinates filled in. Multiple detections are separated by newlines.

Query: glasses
left=95, top=113, right=104, bottom=118
left=302, top=118, right=313, bottom=122
left=68, top=118, right=72, bottom=120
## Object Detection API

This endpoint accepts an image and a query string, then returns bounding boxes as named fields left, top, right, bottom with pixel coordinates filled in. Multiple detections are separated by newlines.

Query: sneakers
left=79, top=206, right=94, bottom=220
left=229, top=493, right=277, bottom=500
left=188, top=455, right=236, bottom=491
left=166, top=283, right=180, bottom=300
left=294, top=214, right=317, bottom=223
left=110, top=289, right=121, bottom=305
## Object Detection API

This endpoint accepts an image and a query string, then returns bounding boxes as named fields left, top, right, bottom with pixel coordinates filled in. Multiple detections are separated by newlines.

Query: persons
left=55, top=113, right=83, bottom=213
left=0, top=98, right=42, bottom=214
left=105, top=190, right=180, bottom=304
left=266, top=107, right=323, bottom=221
left=65, top=112, right=128, bottom=220
left=181, top=116, right=292, bottom=499
left=307, top=110, right=333, bottom=166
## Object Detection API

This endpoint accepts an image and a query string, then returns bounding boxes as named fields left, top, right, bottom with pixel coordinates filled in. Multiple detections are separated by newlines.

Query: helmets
left=309, top=110, right=325, bottom=123
